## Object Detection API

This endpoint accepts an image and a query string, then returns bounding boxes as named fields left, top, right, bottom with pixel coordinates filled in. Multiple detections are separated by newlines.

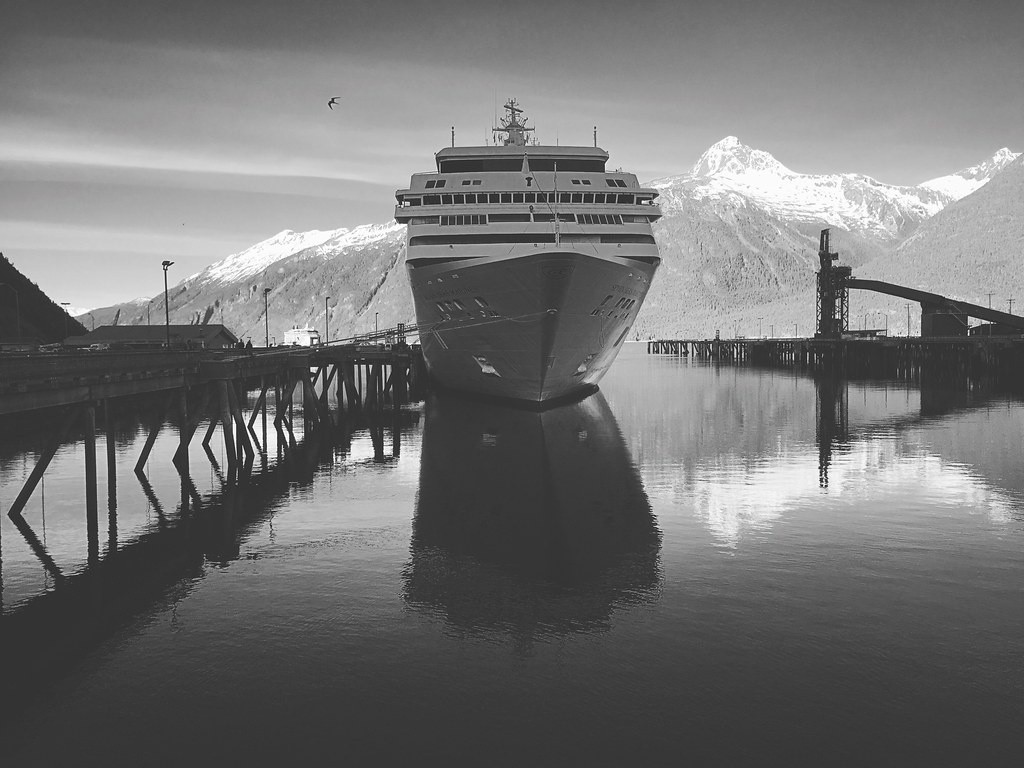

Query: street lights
left=376, top=312, right=378, bottom=345
left=162, top=260, right=174, bottom=352
left=325, top=296, right=330, bottom=347
left=60, top=302, right=70, bottom=338
left=0, top=282, right=21, bottom=339
left=263, top=287, right=272, bottom=348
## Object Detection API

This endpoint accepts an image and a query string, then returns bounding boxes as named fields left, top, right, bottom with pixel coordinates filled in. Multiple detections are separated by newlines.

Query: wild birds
left=328, top=97, right=340, bottom=110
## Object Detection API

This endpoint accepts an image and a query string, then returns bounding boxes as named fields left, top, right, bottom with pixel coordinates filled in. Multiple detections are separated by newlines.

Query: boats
left=401, top=379, right=663, bottom=629
left=394, top=97, right=662, bottom=405
